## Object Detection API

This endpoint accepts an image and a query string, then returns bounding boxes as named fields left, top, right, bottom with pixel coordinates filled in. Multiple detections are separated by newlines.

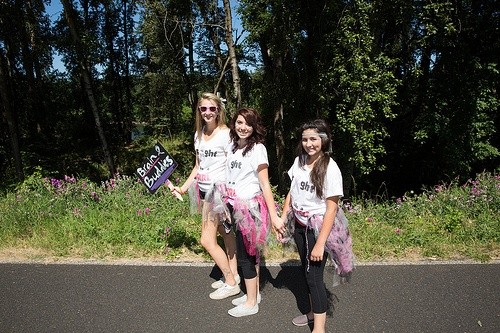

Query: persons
left=225, top=106, right=286, bottom=317
left=170, top=89, right=241, bottom=300
left=275, top=119, right=345, bottom=333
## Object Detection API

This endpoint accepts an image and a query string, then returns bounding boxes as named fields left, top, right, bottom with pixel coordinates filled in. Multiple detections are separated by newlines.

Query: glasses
left=198, top=106, right=219, bottom=111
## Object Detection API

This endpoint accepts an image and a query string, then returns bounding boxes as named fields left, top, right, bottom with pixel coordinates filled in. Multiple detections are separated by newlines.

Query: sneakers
left=232, top=294, right=261, bottom=306
left=292, top=314, right=314, bottom=326
left=211, top=274, right=241, bottom=288
left=228, top=303, right=258, bottom=317
left=209, top=284, right=241, bottom=300
